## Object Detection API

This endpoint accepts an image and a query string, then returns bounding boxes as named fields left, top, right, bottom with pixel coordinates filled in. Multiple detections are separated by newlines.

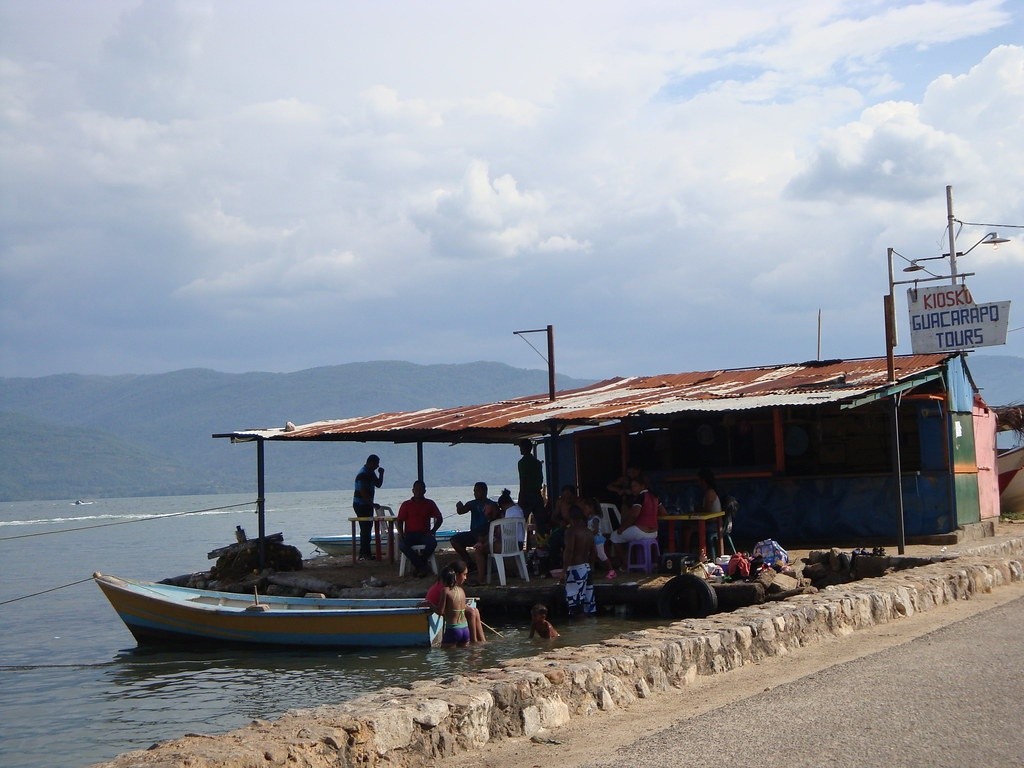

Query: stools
left=628, top=538, right=662, bottom=577
left=400, top=545, right=439, bottom=578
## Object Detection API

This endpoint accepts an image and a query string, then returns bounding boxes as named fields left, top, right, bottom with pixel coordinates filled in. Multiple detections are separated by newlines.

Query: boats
left=93, top=570, right=481, bottom=650
left=995, top=444, right=1024, bottom=515
left=307, top=527, right=472, bottom=557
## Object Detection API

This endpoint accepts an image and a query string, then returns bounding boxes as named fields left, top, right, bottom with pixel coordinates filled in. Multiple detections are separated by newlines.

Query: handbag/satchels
left=728, top=537, right=789, bottom=580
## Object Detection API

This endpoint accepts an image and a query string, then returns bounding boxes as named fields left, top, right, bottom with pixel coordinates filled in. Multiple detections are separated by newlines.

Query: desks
left=348, top=517, right=400, bottom=562
left=658, top=511, right=725, bottom=563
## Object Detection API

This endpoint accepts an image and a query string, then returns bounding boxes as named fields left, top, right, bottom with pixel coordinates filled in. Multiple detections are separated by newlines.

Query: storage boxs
left=661, top=553, right=698, bottom=576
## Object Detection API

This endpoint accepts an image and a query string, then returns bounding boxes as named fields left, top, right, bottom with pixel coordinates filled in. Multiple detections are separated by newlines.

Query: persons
left=472, top=489, right=525, bottom=586
left=529, top=604, right=558, bottom=638
left=353, top=455, right=385, bottom=560
left=398, top=480, right=443, bottom=578
left=450, top=482, right=499, bottom=574
left=684, top=470, right=723, bottom=554
left=606, top=466, right=668, bottom=572
left=421, top=567, right=470, bottom=648
left=564, top=506, right=598, bottom=618
left=553, top=487, right=616, bottom=580
left=517, top=438, right=548, bottom=538
left=426, top=563, right=486, bottom=642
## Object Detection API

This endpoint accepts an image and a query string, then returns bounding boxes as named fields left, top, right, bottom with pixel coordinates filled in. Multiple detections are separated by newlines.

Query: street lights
left=901, top=232, right=1011, bottom=286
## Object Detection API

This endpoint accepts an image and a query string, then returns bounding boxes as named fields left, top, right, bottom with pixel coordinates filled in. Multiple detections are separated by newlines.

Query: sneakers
left=604, top=569, right=617, bottom=580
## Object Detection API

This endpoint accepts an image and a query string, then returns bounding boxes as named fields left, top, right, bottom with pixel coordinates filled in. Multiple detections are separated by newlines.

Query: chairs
left=486, top=517, right=531, bottom=586
left=593, top=503, right=621, bottom=557
left=375, top=506, right=404, bottom=561
left=707, top=497, right=738, bottom=560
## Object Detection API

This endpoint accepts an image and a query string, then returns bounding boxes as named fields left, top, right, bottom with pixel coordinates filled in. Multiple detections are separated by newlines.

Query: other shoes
left=478, top=576, right=486, bottom=585
left=879, top=547, right=885, bottom=556
left=618, top=567, right=627, bottom=573
left=413, top=567, right=419, bottom=577
left=464, top=574, right=478, bottom=583
left=873, top=547, right=880, bottom=556
